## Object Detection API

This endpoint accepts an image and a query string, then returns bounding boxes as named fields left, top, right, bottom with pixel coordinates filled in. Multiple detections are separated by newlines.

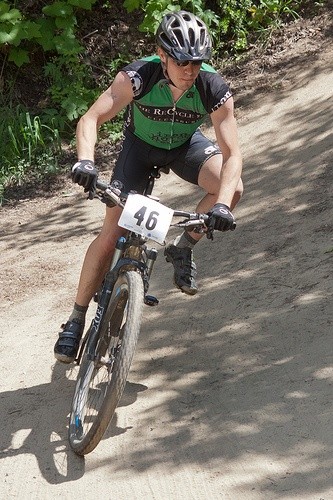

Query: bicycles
left=58, top=149, right=237, bottom=454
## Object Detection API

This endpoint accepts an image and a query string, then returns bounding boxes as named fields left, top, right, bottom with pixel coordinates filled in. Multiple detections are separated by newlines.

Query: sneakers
left=164, top=238, right=198, bottom=295
left=54, top=318, right=85, bottom=363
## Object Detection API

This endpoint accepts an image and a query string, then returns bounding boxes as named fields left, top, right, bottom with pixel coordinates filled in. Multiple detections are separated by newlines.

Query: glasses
left=174, top=60, right=203, bottom=66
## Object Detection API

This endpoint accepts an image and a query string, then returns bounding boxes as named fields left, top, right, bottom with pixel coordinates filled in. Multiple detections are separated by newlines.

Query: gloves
left=72, top=160, right=98, bottom=200
left=204, top=203, right=234, bottom=239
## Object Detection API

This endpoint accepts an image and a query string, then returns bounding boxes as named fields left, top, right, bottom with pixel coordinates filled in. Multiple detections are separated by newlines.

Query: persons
left=52, top=11, right=245, bottom=364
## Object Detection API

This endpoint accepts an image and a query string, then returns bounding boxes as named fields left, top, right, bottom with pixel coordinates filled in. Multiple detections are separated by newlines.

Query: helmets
left=156, top=11, right=212, bottom=61
